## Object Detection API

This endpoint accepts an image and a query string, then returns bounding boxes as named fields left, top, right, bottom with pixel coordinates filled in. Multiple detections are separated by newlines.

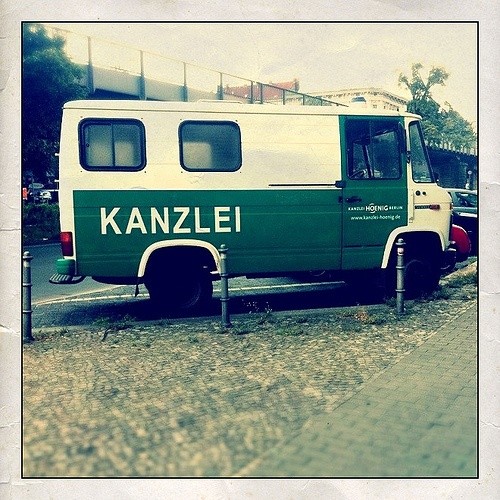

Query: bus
left=48, top=98, right=470, bottom=311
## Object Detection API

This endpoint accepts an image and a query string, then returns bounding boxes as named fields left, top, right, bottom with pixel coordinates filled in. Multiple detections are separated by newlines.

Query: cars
left=450, top=210, right=477, bottom=250
left=438, top=187, right=477, bottom=212
